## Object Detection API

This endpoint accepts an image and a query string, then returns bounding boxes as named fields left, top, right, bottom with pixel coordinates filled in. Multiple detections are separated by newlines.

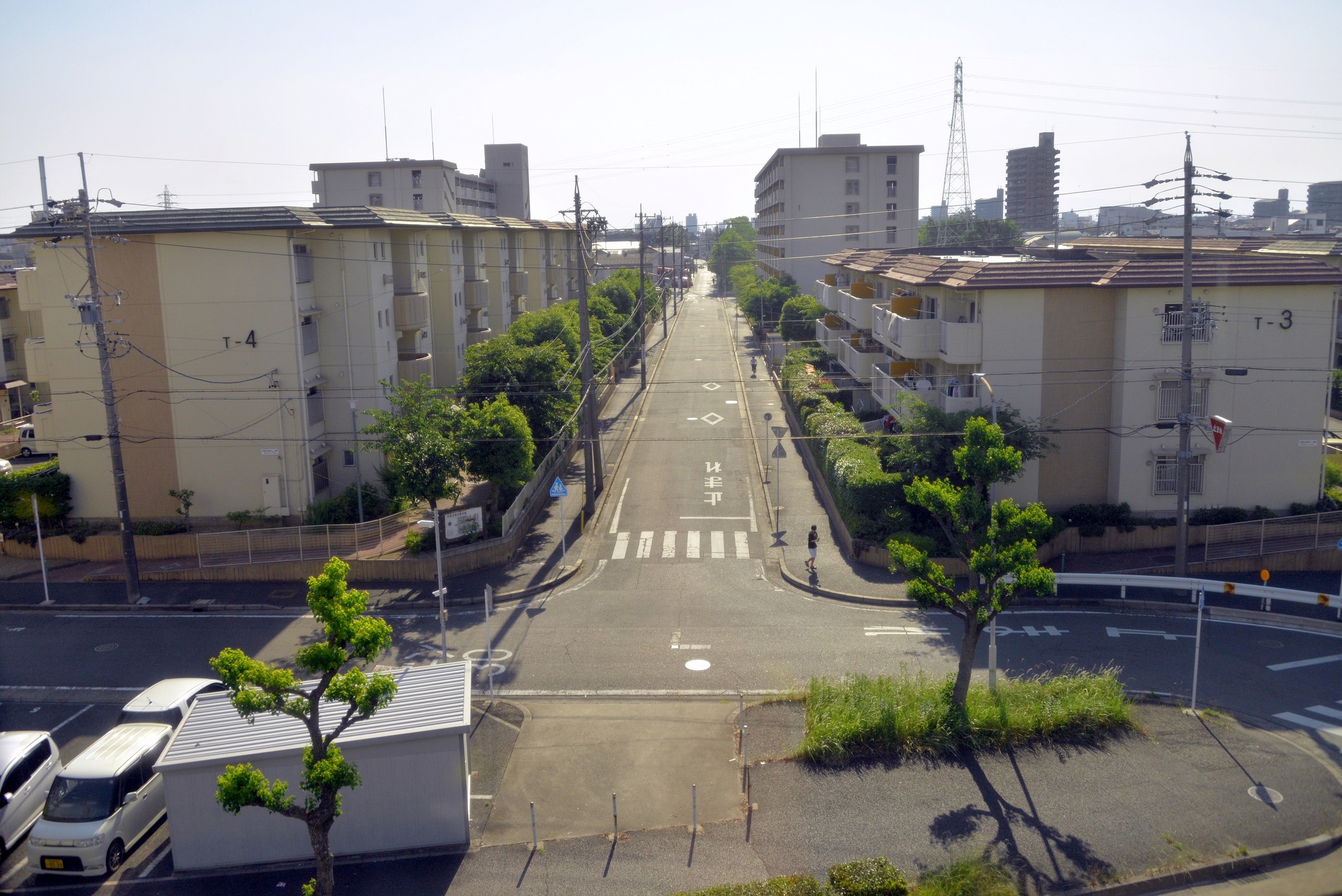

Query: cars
left=0, top=730, right=64, bottom=860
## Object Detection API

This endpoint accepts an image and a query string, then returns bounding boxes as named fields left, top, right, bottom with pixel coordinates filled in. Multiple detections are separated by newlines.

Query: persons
left=805, top=525, right=820, bottom=569
left=750, top=356, right=758, bottom=373
left=712, top=278, right=715, bottom=284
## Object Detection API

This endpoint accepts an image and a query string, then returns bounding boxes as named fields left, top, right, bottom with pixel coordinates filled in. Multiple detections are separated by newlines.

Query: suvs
left=0, top=458, right=13, bottom=478
left=18, top=424, right=39, bottom=457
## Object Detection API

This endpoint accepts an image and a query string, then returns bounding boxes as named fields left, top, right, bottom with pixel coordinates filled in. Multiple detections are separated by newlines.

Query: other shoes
left=805, top=560, right=810, bottom=567
left=810, top=566, right=817, bottom=569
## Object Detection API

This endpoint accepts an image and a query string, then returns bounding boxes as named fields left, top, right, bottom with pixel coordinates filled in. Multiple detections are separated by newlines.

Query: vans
left=114, top=678, right=232, bottom=731
left=27, top=722, right=175, bottom=876
left=672, top=277, right=693, bottom=288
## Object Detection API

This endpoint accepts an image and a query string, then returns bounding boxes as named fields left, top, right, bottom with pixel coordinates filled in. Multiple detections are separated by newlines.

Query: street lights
left=417, top=507, right=447, bottom=664
left=973, top=373, right=996, bottom=671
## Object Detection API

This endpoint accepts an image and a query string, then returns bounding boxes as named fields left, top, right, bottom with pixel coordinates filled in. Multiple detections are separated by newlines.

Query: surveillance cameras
left=432, top=588, right=447, bottom=597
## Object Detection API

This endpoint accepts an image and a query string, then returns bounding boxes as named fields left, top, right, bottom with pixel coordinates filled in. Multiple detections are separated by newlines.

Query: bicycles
left=752, top=362, right=756, bottom=375
